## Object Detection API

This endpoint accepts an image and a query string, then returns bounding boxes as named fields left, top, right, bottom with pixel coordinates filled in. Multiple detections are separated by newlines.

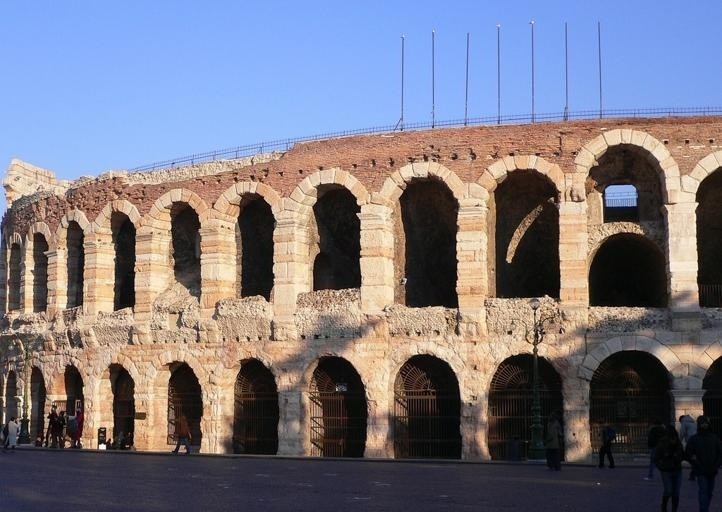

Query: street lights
left=18, top=338, right=32, bottom=444
left=520, top=297, right=556, bottom=459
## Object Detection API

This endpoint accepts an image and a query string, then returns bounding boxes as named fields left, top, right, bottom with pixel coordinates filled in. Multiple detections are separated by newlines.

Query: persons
left=640, top=354, right=722, bottom=511
left=594, top=418, right=616, bottom=471
left=544, top=410, right=564, bottom=473
left=170, top=413, right=193, bottom=454
left=0, top=416, right=20, bottom=451
left=43, top=404, right=84, bottom=449
